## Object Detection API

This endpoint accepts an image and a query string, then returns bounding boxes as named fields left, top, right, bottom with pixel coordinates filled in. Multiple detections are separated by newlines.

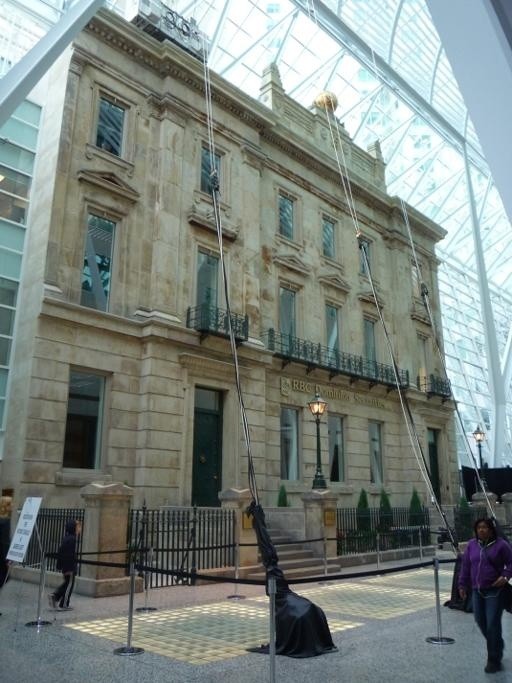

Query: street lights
left=472, top=427, right=489, bottom=491
left=309, top=393, right=327, bottom=489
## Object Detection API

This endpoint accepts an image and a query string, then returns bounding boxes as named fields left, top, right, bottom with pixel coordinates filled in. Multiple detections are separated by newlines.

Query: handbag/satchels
left=502, top=582, right=512, bottom=613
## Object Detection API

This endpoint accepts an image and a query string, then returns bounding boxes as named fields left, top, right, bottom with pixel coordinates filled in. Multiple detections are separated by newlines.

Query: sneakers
left=48, top=591, right=73, bottom=611
left=482, top=639, right=505, bottom=673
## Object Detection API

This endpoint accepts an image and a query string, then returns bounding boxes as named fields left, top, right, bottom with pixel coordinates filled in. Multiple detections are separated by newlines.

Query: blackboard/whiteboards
left=5, top=497, right=43, bottom=564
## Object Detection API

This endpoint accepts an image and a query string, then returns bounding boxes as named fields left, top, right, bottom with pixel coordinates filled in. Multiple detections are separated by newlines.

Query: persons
left=46, top=519, right=82, bottom=613
left=1, top=494, right=15, bottom=588
left=454, top=517, right=512, bottom=673
